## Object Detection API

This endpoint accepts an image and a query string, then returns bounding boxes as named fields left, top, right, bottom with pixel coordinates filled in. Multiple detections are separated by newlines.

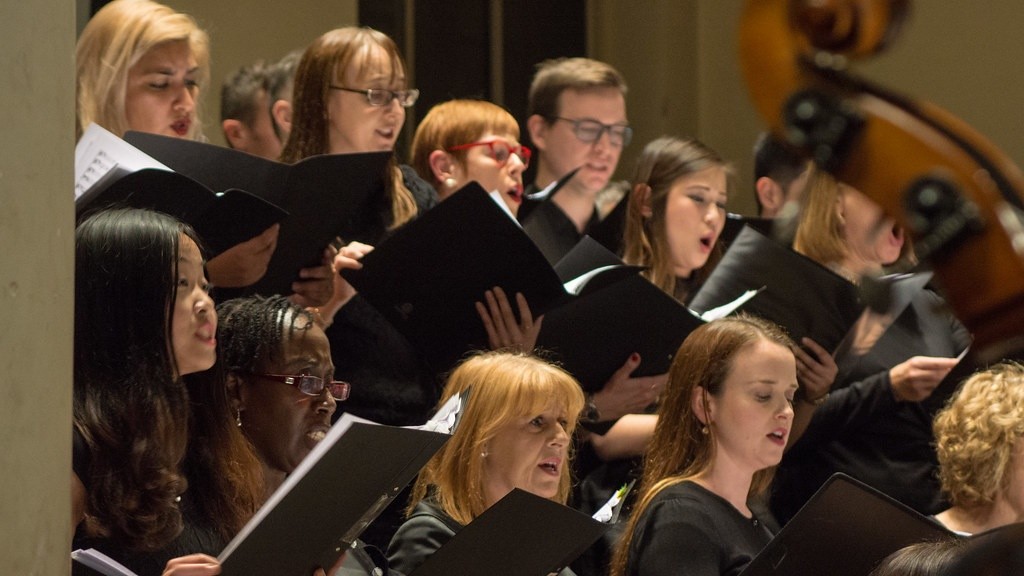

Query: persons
left=411, top=100, right=671, bottom=421
left=75, top=0, right=336, bottom=306
left=609, top=315, right=799, bottom=576
left=138, top=296, right=387, bottom=576
left=752, top=130, right=975, bottom=434
left=75, top=209, right=347, bottom=576
left=929, top=360, right=1024, bottom=537
left=383, top=352, right=586, bottom=575
left=870, top=542, right=977, bottom=576
left=527, top=58, right=630, bottom=262
left=627, top=135, right=838, bottom=448
left=219, top=26, right=544, bottom=352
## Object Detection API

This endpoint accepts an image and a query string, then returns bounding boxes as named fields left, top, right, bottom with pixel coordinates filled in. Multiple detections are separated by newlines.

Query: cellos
left=732, top=1, right=1024, bottom=366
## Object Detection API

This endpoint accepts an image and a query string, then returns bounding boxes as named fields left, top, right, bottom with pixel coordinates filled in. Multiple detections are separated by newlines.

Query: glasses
left=232, top=371, right=351, bottom=402
left=444, top=139, right=531, bottom=170
left=553, top=115, right=633, bottom=147
left=326, top=85, right=420, bottom=107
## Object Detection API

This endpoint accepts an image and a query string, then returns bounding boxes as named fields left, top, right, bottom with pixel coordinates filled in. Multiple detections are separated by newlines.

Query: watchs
left=587, top=397, right=600, bottom=421
